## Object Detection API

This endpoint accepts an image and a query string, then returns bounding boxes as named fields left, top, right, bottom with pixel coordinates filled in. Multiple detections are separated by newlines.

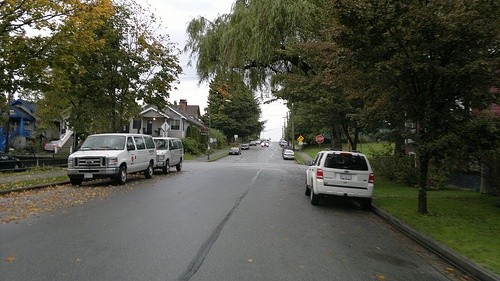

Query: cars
left=255, top=138, right=269, bottom=147
left=249, top=141, right=256, bottom=146
left=44, top=141, right=61, bottom=152
left=280, top=139, right=295, bottom=159
left=241, top=144, right=249, bottom=150
left=229, top=147, right=241, bottom=155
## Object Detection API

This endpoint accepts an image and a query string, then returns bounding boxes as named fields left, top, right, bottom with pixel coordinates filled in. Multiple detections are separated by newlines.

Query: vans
left=152, top=137, right=183, bottom=174
left=67, top=133, right=157, bottom=185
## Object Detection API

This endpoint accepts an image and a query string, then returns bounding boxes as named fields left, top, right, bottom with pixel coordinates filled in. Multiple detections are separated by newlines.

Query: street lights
left=208, top=100, right=231, bottom=160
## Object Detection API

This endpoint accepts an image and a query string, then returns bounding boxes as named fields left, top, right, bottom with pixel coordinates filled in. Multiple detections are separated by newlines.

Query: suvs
left=305, top=148, right=374, bottom=210
